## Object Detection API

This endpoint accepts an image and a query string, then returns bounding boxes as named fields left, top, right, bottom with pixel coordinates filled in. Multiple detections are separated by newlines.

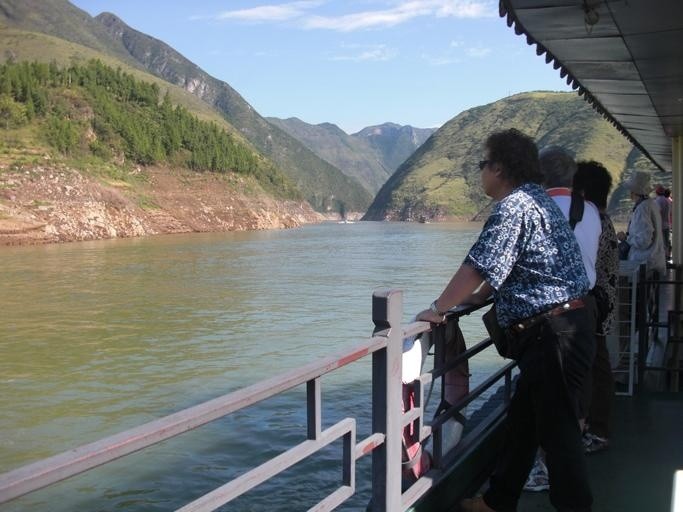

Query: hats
left=620, top=169, right=655, bottom=196
left=656, top=185, right=665, bottom=194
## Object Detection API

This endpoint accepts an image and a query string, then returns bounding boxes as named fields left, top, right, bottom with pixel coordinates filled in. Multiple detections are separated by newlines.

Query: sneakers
left=581, top=433, right=608, bottom=455
left=521, top=455, right=554, bottom=492
left=458, top=497, right=494, bottom=512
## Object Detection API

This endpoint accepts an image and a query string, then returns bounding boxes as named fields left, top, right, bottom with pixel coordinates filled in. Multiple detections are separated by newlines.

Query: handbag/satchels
left=618, top=240, right=630, bottom=258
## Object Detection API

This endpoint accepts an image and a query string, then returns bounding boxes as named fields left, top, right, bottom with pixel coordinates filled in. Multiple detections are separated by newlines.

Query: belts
left=511, top=298, right=582, bottom=333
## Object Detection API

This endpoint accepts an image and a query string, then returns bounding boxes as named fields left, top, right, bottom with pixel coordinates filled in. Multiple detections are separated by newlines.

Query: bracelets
left=430, top=300, right=445, bottom=316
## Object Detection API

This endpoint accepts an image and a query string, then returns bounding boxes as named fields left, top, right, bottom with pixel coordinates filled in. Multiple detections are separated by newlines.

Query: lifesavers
left=402, top=317, right=468, bottom=486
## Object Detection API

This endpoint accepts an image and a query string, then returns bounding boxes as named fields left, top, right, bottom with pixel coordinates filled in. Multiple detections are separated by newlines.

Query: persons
left=616, top=171, right=667, bottom=282
left=517, top=144, right=604, bottom=496
left=413, top=126, right=601, bottom=510
left=663, top=189, right=672, bottom=227
left=568, top=160, right=622, bottom=455
left=653, top=183, right=671, bottom=262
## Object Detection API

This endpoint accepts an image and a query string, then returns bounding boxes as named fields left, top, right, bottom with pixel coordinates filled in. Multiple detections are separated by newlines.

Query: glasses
left=479, top=160, right=492, bottom=171
